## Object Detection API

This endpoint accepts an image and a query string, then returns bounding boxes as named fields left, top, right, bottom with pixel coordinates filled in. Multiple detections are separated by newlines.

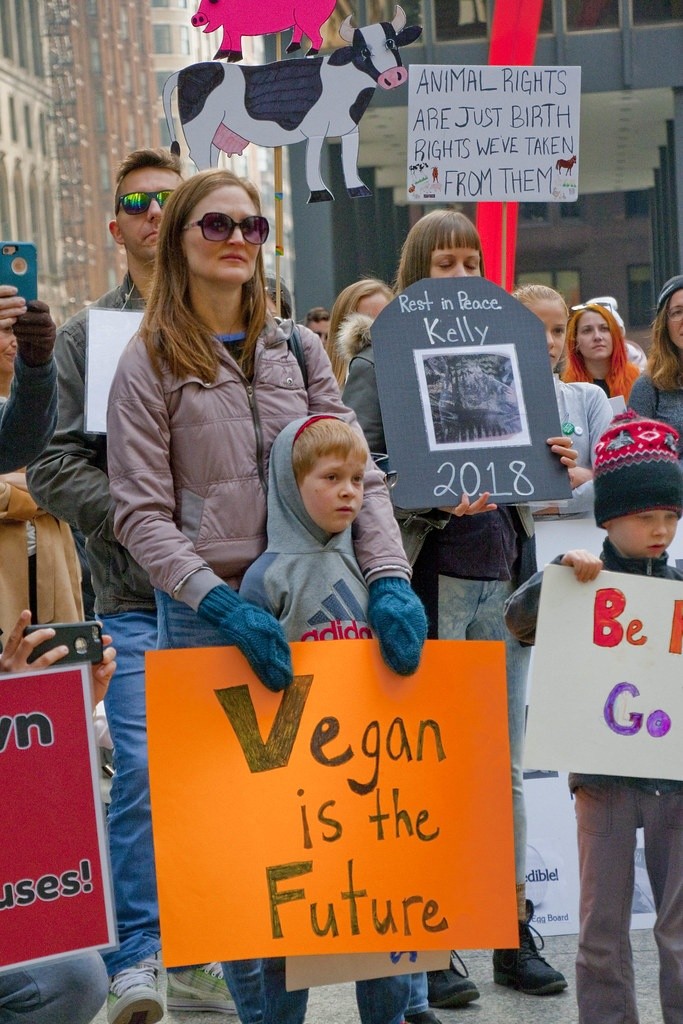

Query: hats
left=656, top=274, right=683, bottom=316
left=594, top=408, right=683, bottom=529
left=263, top=278, right=292, bottom=318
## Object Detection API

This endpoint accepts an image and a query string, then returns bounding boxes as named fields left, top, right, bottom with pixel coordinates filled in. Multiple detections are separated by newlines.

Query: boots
left=493, top=899, right=568, bottom=994
left=427, top=950, right=480, bottom=1008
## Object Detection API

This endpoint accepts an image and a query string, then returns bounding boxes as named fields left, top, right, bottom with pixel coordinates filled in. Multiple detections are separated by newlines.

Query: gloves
left=13, top=300, right=56, bottom=369
left=198, top=584, right=293, bottom=692
left=367, top=578, right=427, bottom=676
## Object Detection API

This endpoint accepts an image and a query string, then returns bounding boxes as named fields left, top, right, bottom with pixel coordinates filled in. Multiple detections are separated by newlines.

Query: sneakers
left=167, top=961, right=238, bottom=1015
left=107, top=953, right=164, bottom=1024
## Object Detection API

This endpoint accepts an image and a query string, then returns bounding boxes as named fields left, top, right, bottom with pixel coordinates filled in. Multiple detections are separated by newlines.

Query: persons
left=0, top=328, right=86, bottom=655
left=561, top=304, right=640, bottom=406
left=105, top=172, right=239, bottom=1024
left=259, top=276, right=292, bottom=320
left=510, top=284, right=614, bottom=523
left=342, top=207, right=578, bottom=1009
left=588, top=295, right=649, bottom=374
left=236, top=414, right=443, bottom=1024
left=323, top=279, right=396, bottom=396
left=502, top=410, right=683, bottom=1024
left=28, top=148, right=188, bottom=1024
left=305, top=305, right=334, bottom=347
left=626, top=273, right=683, bottom=459
left=0, top=608, right=117, bottom=1024
left=0, top=285, right=59, bottom=476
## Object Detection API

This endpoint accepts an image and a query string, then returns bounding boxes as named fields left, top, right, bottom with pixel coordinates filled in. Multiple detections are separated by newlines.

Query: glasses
left=571, top=302, right=612, bottom=318
left=666, top=307, right=683, bottom=321
left=115, top=189, right=176, bottom=215
left=180, top=212, right=269, bottom=245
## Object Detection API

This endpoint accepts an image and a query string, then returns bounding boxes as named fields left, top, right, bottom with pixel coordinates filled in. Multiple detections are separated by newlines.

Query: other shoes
left=405, top=1008, right=442, bottom=1024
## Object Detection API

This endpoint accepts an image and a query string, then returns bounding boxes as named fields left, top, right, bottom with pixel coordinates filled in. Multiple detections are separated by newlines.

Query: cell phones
left=0, top=242, right=38, bottom=304
left=21, top=621, right=104, bottom=666
